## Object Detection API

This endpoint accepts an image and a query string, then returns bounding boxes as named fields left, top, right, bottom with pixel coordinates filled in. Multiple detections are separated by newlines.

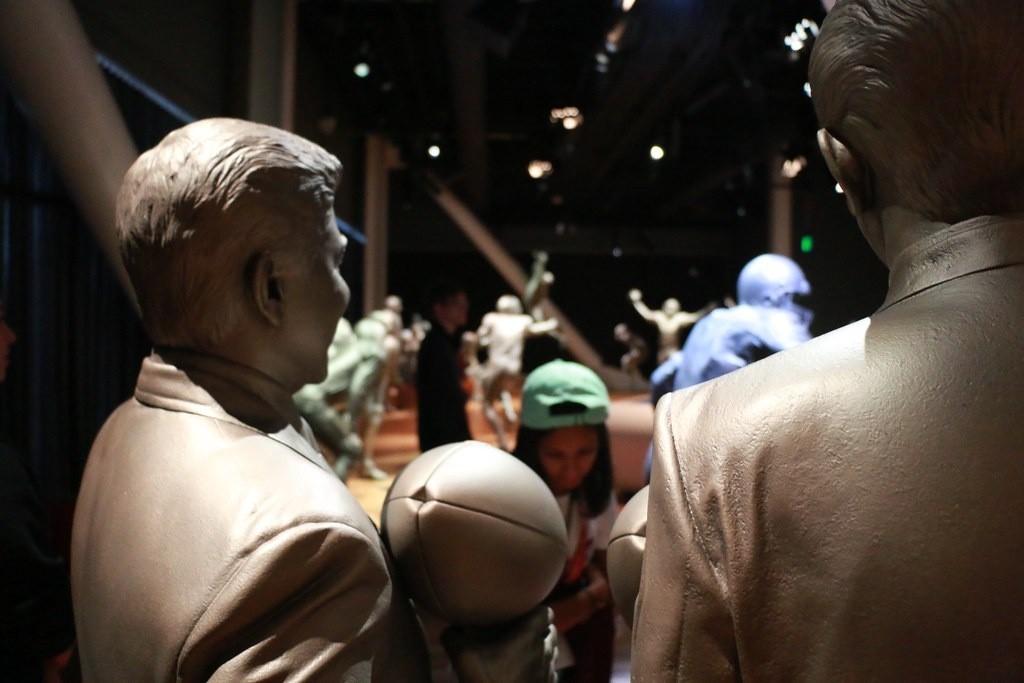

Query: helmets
left=738, top=254, right=812, bottom=330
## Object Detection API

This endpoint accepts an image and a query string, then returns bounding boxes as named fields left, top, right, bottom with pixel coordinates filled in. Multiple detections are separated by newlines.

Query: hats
left=520, top=360, right=609, bottom=429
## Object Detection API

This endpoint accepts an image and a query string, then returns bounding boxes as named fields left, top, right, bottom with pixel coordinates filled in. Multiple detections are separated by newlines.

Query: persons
left=611, top=0, right=1024, bottom=683
left=509, top=360, right=622, bottom=683
left=70, top=116, right=567, bottom=683
left=413, top=282, right=477, bottom=453
